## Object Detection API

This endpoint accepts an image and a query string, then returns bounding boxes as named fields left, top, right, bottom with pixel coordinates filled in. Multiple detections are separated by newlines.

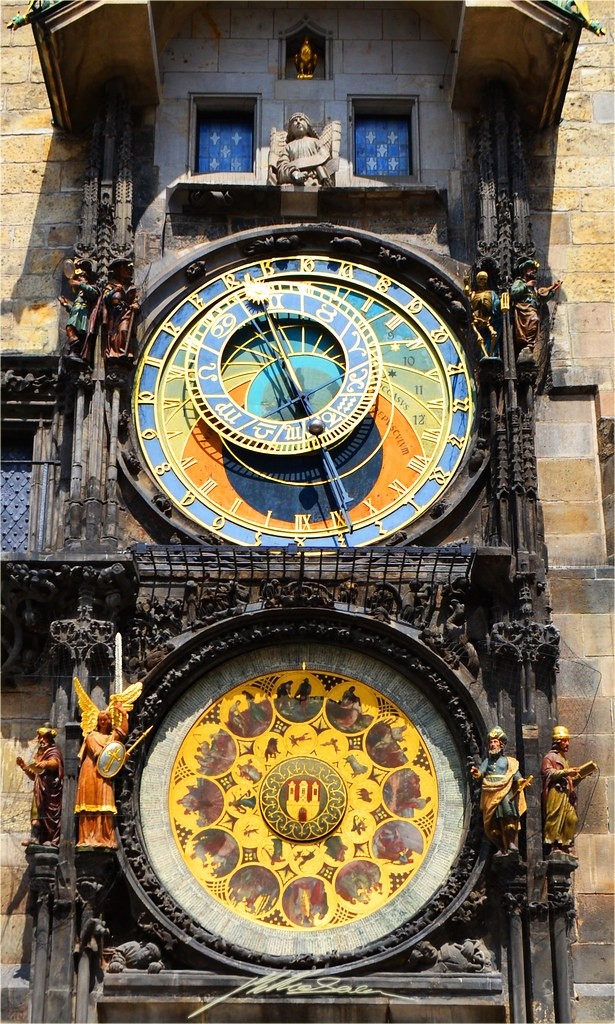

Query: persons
left=16, top=728, right=63, bottom=845
left=101, top=263, right=140, bottom=357
left=408, top=941, right=484, bottom=971
left=106, top=940, right=165, bottom=973
left=510, top=260, right=563, bottom=350
left=278, top=113, right=329, bottom=185
left=470, top=726, right=531, bottom=855
left=76, top=702, right=128, bottom=847
left=542, top=725, right=579, bottom=860
left=59, top=271, right=99, bottom=346
left=463, top=271, right=499, bottom=358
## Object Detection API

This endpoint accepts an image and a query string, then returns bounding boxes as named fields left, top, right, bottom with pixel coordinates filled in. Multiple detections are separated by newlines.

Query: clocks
left=114, top=220, right=501, bottom=550
